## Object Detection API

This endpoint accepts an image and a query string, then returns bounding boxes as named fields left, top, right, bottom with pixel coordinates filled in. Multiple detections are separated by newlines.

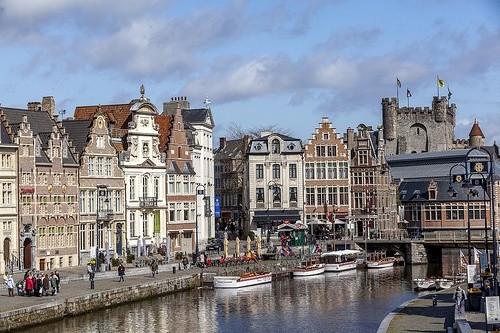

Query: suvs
left=206, top=239, right=224, bottom=251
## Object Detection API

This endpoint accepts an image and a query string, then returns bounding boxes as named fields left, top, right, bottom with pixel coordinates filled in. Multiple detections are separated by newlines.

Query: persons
left=453, top=286, right=467, bottom=313
left=118, top=263, right=125, bottom=282
left=182, top=253, right=224, bottom=270
left=150, top=261, right=159, bottom=277
left=3, top=269, right=61, bottom=297
left=87, top=264, right=96, bottom=290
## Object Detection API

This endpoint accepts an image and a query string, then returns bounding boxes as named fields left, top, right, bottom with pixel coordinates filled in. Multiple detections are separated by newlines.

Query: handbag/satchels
left=462, top=290, right=465, bottom=300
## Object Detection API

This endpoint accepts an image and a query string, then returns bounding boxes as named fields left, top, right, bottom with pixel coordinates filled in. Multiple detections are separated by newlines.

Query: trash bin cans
left=172, top=267, right=176, bottom=274
left=101, top=264, right=107, bottom=271
left=467, top=288, right=483, bottom=312
left=481, top=273, right=492, bottom=291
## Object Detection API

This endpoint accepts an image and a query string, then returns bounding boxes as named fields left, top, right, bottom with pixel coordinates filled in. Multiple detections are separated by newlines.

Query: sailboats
left=443, top=246, right=484, bottom=279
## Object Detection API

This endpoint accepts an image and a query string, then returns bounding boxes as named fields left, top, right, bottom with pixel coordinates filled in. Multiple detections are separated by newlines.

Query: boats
left=292, top=264, right=326, bottom=276
left=413, top=277, right=436, bottom=291
left=435, top=279, right=463, bottom=290
left=321, top=249, right=363, bottom=272
left=366, top=256, right=396, bottom=267
left=213, top=271, right=272, bottom=288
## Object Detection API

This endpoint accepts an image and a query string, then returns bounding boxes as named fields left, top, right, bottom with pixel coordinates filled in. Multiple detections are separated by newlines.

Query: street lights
left=195, top=184, right=208, bottom=267
left=95, top=184, right=109, bottom=273
left=363, top=187, right=376, bottom=240
left=267, top=180, right=278, bottom=242
left=460, top=146, right=498, bottom=296
left=447, top=163, right=472, bottom=265
